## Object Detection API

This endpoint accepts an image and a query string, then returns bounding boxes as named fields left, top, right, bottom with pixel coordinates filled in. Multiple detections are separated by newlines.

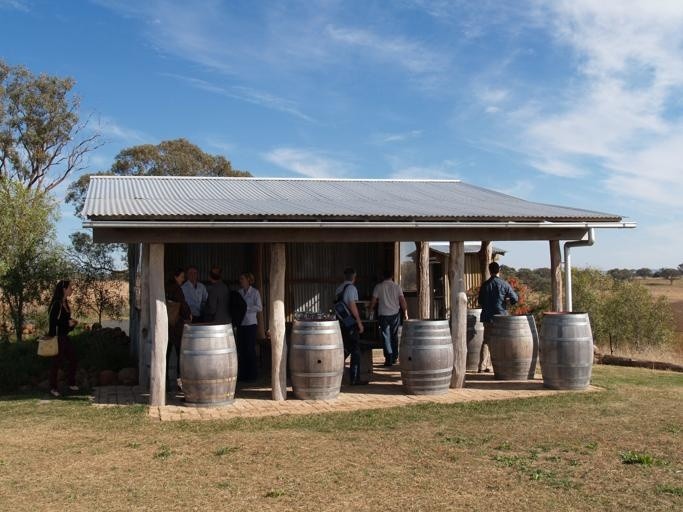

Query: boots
left=349, top=359, right=369, bottom=386
left=67, top=359, right=80, bottom=394
left=48, top=365, right=62, bottom=398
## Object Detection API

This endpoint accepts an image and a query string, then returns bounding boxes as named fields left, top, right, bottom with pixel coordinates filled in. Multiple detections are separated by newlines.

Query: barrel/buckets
left=288, top=319, right=345, bottom=400
left=178, top=321, right=239, bottom=407
left=467, top=308, right=490, bottom=371
left=489, top=315, right=538, bottom=381
left=399, top=319, right=455, bottom=395
left=539, top=311, right=594, bottom=390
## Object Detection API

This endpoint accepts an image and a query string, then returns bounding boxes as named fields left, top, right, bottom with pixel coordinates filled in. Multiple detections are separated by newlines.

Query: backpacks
left=332, top=283, right=361, bottom=332
left=223, top=279, right=248, bottom=328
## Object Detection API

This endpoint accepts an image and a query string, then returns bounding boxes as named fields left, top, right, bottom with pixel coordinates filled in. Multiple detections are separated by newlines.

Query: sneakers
left=477, top=369, right=490, bottom=373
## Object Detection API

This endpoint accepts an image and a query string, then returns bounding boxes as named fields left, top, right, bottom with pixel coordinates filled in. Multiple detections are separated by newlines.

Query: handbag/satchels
left=36, top=335, right=59, bottom=358
left=168, top=300, right=181, bottom=326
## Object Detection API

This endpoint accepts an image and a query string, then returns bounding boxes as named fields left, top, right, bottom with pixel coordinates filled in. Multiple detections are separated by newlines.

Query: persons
left=367, top=268, right=408, bottom=366
left=162, top=261, right=265, bottom=395
left=47, top=280, right=80, bottom=397
left=477, top=262, right=519, bottom=373
left=333, top=266, right=368, bottom=386
